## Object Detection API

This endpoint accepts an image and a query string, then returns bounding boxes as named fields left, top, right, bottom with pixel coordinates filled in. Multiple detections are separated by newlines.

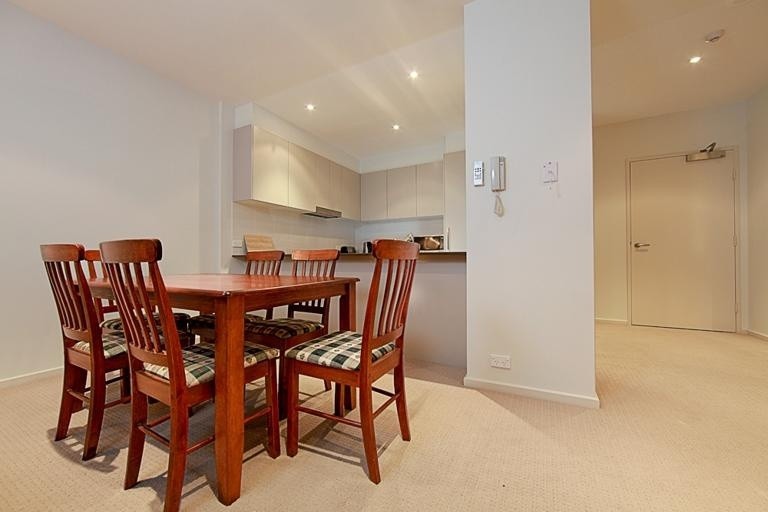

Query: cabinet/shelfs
left=232, top=124, right=361, bottom=224
left=442, top=150, right=468, bottom=250
left=361, top=161, right=444, bottom=225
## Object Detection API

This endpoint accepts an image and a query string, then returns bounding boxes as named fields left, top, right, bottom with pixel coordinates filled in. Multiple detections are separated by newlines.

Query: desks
left=59, top=272, right=361, bottom=505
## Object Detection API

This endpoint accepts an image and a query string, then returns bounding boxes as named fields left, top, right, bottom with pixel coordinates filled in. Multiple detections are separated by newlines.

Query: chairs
left=85, top=249, right=191, bottom=405
left=189, top=250, right=284, bottom=339
left=285, top=238, right=420, bottom=485
left=40, top=242, right=189, bottom=462
left=99, top=239, right=281, bottom=511
left=244, top=249, right=340, bottom=418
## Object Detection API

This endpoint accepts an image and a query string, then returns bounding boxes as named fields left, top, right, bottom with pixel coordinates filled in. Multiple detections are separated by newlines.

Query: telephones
left=492, top=157, right=504, bottom=192
